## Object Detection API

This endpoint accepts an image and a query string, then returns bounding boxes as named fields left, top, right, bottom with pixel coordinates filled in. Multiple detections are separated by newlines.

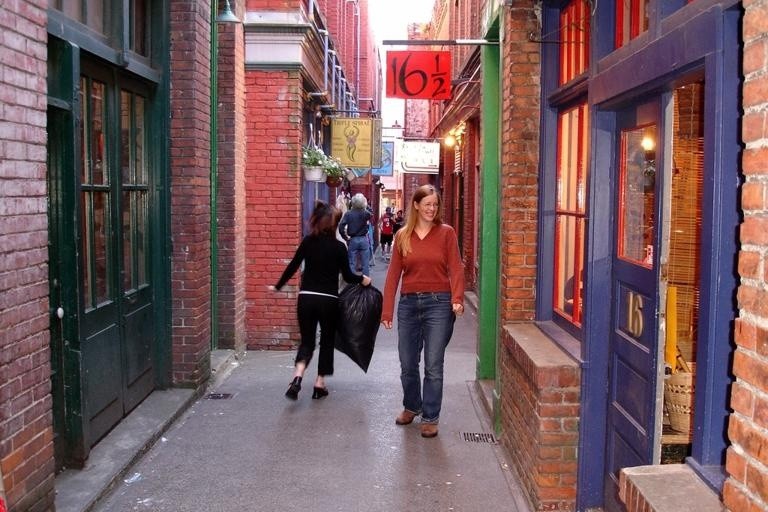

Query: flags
left=303, top=166, right=344, bottom=188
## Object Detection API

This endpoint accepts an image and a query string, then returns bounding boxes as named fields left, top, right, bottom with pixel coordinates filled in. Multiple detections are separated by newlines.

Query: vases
left=301, top=145, right=345, bottom=177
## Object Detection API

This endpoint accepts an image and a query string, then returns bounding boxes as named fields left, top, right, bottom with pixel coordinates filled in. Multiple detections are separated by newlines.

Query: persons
left=390, top=203, right=397, bottom=219
left=379, top=207, right=396, bottom=256
left=381, top=184, right=466, bottom=438
left=392, top=210, right=405, bottom=235
left=268, top=200, right=372, bottom=400
left=339, top=193, right=374, bottom=276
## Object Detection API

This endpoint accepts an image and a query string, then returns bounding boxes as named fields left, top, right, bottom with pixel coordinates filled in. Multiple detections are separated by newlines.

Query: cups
left=417, top=202, right=439, bottom=208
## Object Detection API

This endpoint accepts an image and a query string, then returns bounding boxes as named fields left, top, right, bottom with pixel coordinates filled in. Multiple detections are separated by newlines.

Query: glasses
left=214, top=0, right=242, bottom=24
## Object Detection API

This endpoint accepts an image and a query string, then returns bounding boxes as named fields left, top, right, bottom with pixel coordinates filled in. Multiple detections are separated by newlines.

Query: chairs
left=421, top=421, right=437, bottom=438
left=395, top=409, right=417, bottom=426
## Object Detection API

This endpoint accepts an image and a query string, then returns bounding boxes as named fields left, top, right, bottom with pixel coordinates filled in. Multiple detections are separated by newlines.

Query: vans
left=285, top=375, right=301, bottom=400
left=311, top=386, right=328, bottom=399
